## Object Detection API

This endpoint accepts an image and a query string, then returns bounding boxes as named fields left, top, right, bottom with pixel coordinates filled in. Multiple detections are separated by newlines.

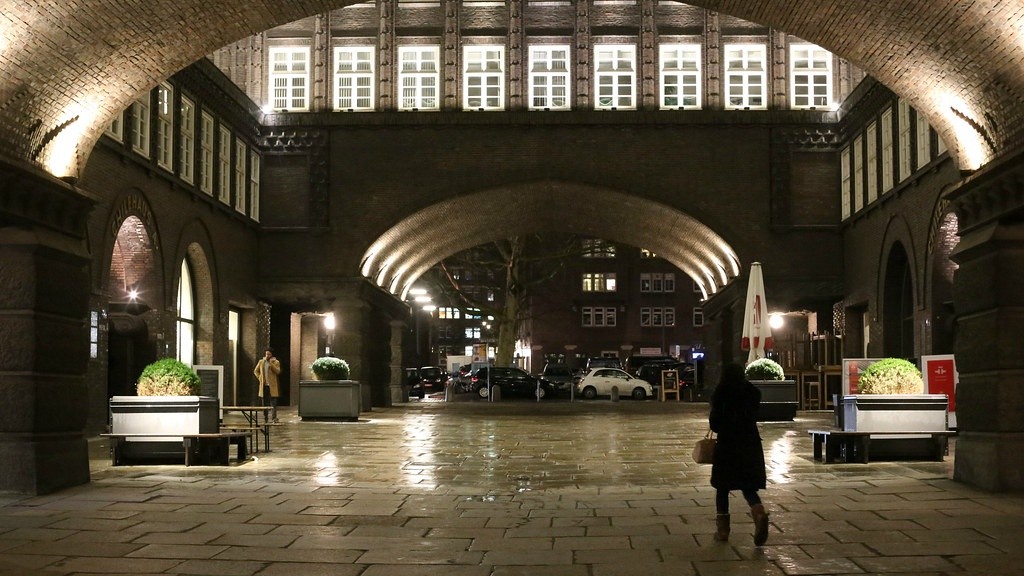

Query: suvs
left=537, top=362, right=575, bottom=397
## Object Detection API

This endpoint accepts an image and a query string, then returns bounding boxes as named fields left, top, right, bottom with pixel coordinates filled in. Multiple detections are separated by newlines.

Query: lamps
left=478, top=108, right=484, bottom=111
left=59, top=176, right=79, bottom=187
left=611, top=106, right=617, bottom=111
left=413, top=108, right=418, bottom=111
left=347, top=108, right=353, bottom=113
left=744, top=106, right=750, bottom=110
left=960, top=169, right=975, bottom=177
left=544, top=108, right=550, bottom=111
left=678, top=106, right=684, bottom=110
left=809, top=106, right=816, bottom=110
left=282, top=108, right=288, bottom=113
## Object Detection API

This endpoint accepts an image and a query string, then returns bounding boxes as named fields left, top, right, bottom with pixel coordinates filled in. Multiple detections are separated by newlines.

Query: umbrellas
left=741, top=261, right=775, bottom=366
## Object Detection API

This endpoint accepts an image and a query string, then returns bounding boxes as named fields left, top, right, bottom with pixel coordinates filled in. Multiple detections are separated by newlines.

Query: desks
left=219, top=405, right=275, bottom=454
left=774, top=328, right=842, bottom=411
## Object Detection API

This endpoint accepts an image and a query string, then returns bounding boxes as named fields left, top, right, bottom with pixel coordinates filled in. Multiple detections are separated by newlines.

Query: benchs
left=100, top=420, right=285, bottom=471
left=807, top=429, right=956, bottom=463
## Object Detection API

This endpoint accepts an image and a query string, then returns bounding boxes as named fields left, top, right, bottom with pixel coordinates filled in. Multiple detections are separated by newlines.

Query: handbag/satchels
left=692, top=430, right=717, bottom=464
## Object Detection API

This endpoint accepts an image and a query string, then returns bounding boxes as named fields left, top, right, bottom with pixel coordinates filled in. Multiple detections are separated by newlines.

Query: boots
left=751, top=504, right=768, bottom=545
left=713, top=514, right=730, bottom=541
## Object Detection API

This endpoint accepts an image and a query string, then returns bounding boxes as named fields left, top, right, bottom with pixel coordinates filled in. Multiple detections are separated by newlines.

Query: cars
left=421, top=366, right=474, bottom=394
left=405, top=367, right=426, bottom=399
left=578, top=367, right=653, bottom=400
left=469, top=365, right=556, bottom=400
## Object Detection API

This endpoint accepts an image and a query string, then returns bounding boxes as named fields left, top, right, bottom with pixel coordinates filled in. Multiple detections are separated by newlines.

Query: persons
left=254, top=347, right=280, bottom=423
left=708, top=365, right=769, bottom=546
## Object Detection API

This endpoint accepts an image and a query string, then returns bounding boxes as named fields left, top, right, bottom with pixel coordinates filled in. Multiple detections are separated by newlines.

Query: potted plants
left=741, top=357, right=799, bottom=422
left=297, top=355, right=361, bottom=421
left=844, top=356, right=949, bottom=457
left=111, top=355, right=221, bottom=463
